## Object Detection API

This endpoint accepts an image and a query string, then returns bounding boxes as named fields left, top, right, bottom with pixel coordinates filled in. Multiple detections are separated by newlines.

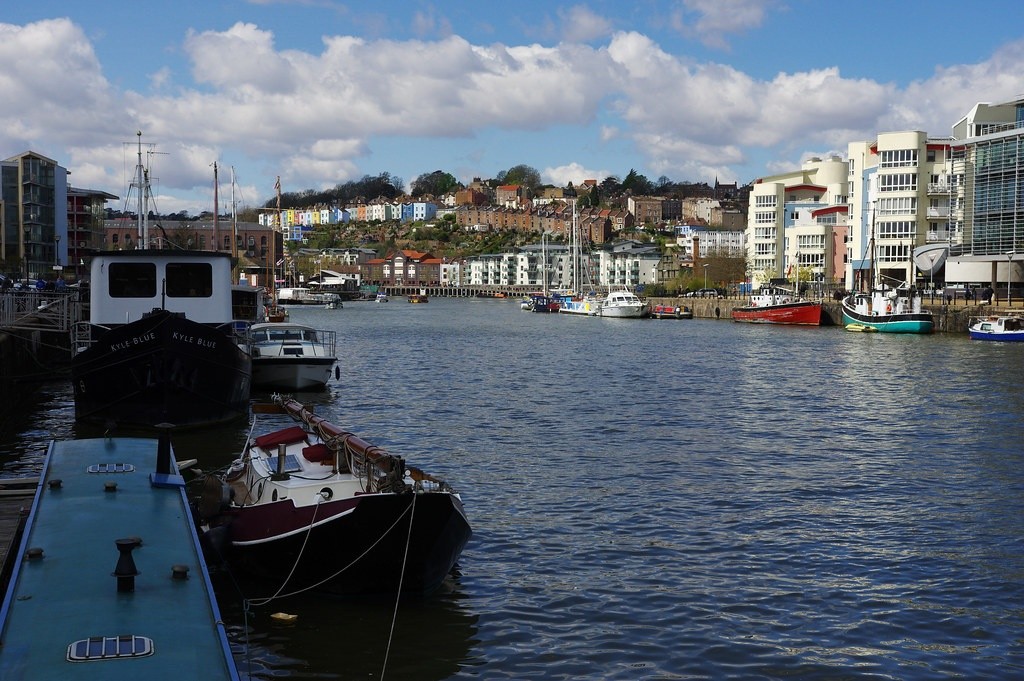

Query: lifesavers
left=886, top=305, right=892, bottom=311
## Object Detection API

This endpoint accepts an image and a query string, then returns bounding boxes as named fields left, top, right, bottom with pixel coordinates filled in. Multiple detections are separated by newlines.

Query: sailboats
left=839, top=208, right=937, bottom=334
left=520, top=199, right=650, bottom=319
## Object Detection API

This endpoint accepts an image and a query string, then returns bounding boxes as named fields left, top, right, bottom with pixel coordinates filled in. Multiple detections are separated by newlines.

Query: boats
left=235, top=319, right=342, bottom=395
left=650, top=303, right=694, bottom=320
left=375, top=292, right=389, bottom=303
left=192, top=388, right=474, bottom=622
left=730, top=277, right=824, bottom=327
left=62, top=126, right=255, bottom=435
left=275, top=287, right=344, bottom=309
left=475, top=290, right=505, bottom=298
left=968, top=311, right=1024, bottom=341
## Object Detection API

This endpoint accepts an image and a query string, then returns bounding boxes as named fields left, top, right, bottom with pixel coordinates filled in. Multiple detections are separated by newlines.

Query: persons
left=965, top=285, right=994, bottom=305
left=947, top=293, right=953, bottom=305
left=918, top=285, right=942, bottom=297
left=1, top=274, right=89, bottom=313
left=832, top=290, right=850, bottom=300
left=717, top=284, right=737, bottom=299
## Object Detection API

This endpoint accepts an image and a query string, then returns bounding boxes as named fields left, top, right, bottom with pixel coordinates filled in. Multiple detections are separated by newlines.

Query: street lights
left=53, top=234, right=61, bottom=276
left=703, top=264, right=710, bottom=290
left=79, top=240, right=86, bottom=279
left=1005, top=250, right=1016, bottom=307
left=23, top=224, right=31, bottom=287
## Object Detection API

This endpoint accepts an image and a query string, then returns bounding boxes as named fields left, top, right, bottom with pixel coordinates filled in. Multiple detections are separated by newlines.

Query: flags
left=786, top=263, right=792, bottom=277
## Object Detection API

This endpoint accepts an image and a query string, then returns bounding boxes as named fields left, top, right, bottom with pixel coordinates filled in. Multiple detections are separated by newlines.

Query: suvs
left=686, top=288, right=718, bottom=297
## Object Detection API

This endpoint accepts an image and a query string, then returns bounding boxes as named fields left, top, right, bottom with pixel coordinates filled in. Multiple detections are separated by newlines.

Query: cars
left=0, top=275, right=46, bottom=288
